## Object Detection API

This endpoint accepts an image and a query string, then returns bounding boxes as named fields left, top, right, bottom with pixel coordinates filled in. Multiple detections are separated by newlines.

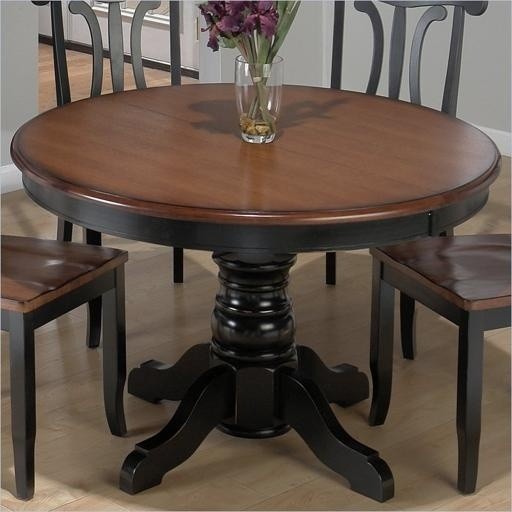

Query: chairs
left=1, top=235, right=128, bottom=499
left=327, top=0, right=488, bottom=289
left=368, top=233, right=511, bottom=494
left=32, top=1, right=183, bottom=284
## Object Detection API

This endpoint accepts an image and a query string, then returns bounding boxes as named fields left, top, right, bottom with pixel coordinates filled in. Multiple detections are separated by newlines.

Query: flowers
left=197, top=0, right=301, bottom=123
